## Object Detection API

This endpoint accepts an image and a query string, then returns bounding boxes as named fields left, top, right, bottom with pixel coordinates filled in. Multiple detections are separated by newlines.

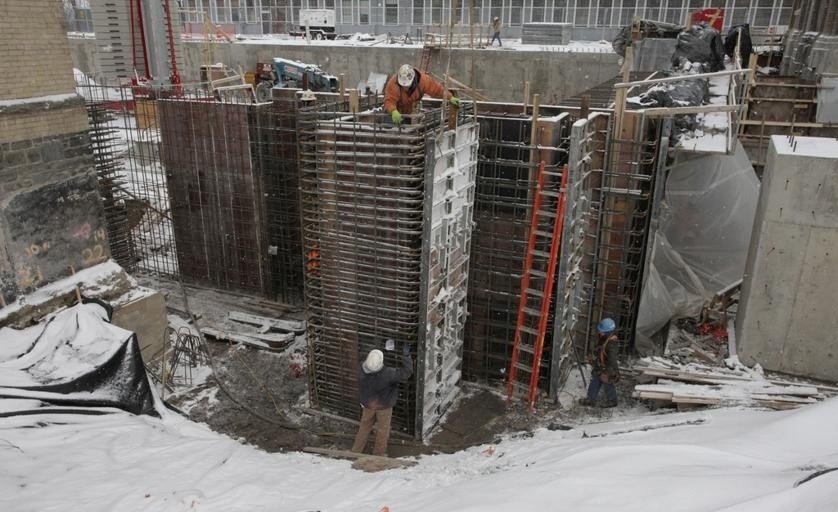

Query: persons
left=577, top=317, right=622, bottom=409
left=490, top=16, right=503, bottom=48
left=350, top=342, right=414, bottom=457
left=381, top=64, right=459, bottom=126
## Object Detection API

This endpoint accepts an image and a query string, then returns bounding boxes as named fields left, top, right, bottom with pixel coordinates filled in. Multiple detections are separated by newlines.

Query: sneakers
left=600, top=400, right=617, bottom=408
left=579, top=398, right=597, bottom=407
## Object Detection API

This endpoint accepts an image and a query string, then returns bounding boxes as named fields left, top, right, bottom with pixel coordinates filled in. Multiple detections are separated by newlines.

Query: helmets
left=398, top=64, right=416, bottom=87
left=494, top=16, right=498, bottom=21
left=366, top=349, right=384, bottom=371
left=597, top=317, right=616, bottom=333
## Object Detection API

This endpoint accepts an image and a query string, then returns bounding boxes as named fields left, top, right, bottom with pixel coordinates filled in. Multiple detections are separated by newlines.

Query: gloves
left=392, top=110, right=404, bottom=125
left=403, top=343, right=410, bottom=353
left=450, top=96, right=460, bottom=106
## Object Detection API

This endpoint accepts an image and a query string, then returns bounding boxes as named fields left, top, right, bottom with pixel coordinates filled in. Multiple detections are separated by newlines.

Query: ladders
left=506, top=160, right=568, bottom=413
